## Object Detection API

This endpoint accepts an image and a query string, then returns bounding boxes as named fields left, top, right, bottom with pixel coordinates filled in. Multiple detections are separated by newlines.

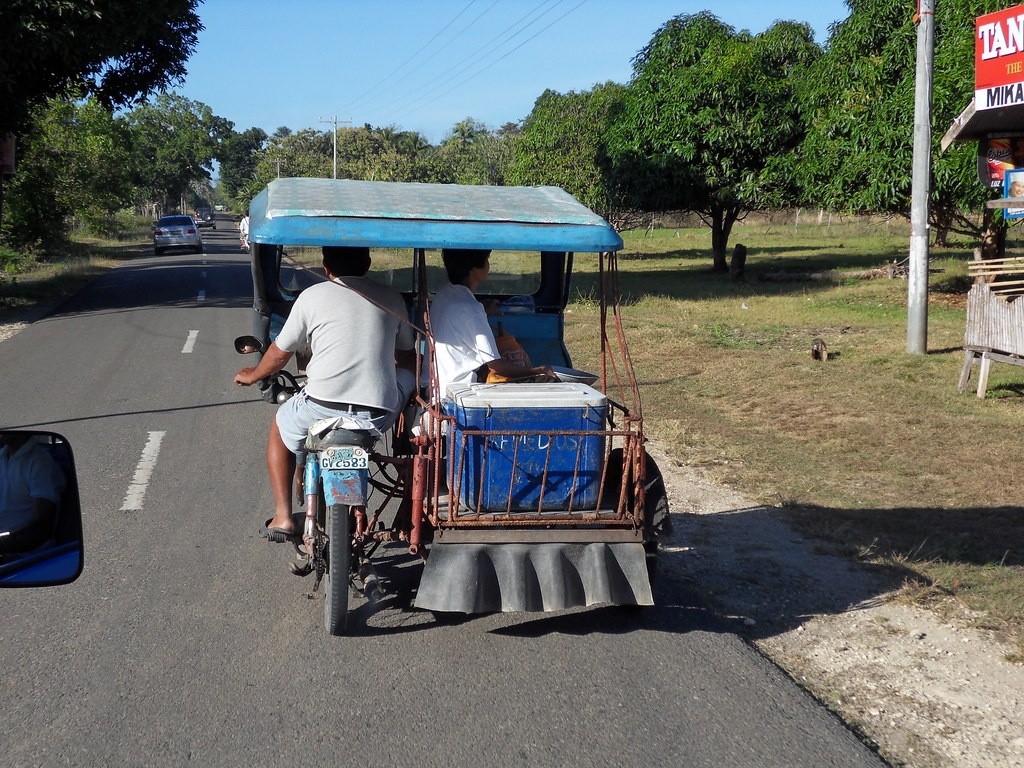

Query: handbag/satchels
left=486, top=336, right=530, bottom=383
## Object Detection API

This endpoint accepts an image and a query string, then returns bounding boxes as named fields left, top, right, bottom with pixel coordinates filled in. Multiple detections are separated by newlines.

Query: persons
left=0, top=432, right=64, bottom=560
left=239, top=339, right=257, bottom=352
left=238, top=210, right=249, bottom=247
left=424, top=249, right=557, bottom=407
left=1007, top=173, right=1024, bottom=197
left=235, top=246, right=423, bottom=536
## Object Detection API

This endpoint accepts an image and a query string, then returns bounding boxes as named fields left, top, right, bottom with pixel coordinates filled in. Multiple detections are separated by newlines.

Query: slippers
left=258, top=517, right=294, bottom=537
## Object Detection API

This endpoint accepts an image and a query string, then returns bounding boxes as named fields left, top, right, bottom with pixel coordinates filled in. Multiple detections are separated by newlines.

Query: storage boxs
left=445, top=382, right=607, bottom=513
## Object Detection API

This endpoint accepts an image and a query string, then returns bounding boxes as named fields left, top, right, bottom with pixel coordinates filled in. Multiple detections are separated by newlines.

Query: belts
left=301, top=389, right=389, bottom=415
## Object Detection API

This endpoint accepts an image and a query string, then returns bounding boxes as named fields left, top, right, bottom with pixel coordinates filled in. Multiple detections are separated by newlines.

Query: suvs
left=195, top=207, right=216, bottom=229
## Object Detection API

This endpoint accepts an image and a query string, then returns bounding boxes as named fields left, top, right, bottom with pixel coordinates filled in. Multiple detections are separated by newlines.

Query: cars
left=150, top=216, right=204, bottom=254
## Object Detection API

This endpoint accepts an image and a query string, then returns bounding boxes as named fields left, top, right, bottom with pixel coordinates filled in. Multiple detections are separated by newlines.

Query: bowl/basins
left=552, top=365, right=600, bottom=386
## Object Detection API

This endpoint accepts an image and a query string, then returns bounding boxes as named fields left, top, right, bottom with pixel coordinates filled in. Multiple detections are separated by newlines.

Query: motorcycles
left=233, top=171, right=676, bottom=639
